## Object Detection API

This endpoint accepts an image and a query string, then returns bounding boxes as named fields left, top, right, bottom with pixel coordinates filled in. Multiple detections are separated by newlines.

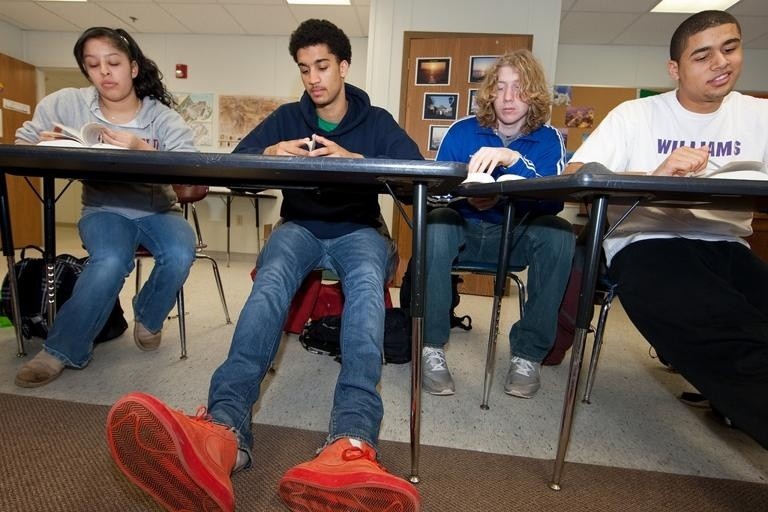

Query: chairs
left=582, top=268, right=618, bottom=404
left=171, top=185, right=209, bottom=254
left=447, top=263, right=527, bottom=320
left=250, top=256, right=398, bottom=335
left=81, top=184, right=232, bottom=361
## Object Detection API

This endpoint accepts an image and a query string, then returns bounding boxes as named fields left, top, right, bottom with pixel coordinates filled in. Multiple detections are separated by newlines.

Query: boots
left=279, top=434, right=419, bottom=512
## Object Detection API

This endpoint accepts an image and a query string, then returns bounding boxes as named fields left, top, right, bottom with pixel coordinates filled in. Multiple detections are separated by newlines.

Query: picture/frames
left=422, top=92, right=459, bottom=122
left=427, top=125, right=453, bottom=152
left=467, top=88, right=479, bottom=117
left=414, top=56, right=452, bottom=87
left=467, top=55, right=504, bottom=85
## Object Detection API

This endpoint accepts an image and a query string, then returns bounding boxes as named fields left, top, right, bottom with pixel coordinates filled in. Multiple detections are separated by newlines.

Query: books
left=462, top=172, right=527, bottom=184
left=35, top=120, right=131, bottom=151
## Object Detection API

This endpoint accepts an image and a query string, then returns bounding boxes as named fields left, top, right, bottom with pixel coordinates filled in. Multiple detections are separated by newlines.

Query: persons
left=104, top=15, right=423, bottom=511
left=11, top=25, right=211, bottom=391
left=563, top=7, right=766, bottom=446
left=415, top=49, right=566, bottom=403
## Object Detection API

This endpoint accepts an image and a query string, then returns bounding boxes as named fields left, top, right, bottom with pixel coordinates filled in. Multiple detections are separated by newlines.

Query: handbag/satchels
left=0, top=245, right=127, bottom=344
left=298, top=308, right=410, bottom=363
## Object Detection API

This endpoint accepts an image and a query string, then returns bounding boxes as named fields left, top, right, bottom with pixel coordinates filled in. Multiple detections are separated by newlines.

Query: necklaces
left=99, top=96, right=140, bottom=122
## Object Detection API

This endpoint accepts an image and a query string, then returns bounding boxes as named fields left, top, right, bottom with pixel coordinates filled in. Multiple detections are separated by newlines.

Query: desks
left=448, top=173, right=768, bottom=491
left=0, top=144, right=467, bottom=485
left=207, top=189, right=277, bottom=268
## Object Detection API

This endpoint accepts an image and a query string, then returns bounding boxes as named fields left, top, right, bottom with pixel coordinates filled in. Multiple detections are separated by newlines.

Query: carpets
left=1, top=391, right=768, bottom=511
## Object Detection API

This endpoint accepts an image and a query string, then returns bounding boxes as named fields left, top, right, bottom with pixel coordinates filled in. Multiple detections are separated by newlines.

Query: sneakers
left=420, top=346, right=454, bottom=396
left=503, top=357, right=540, bottom=399
left=107, top=394, right=237, bottom=512
left=14, top=348, right=65, bottom=389
left=132, top=294, right=161, bottom=352
